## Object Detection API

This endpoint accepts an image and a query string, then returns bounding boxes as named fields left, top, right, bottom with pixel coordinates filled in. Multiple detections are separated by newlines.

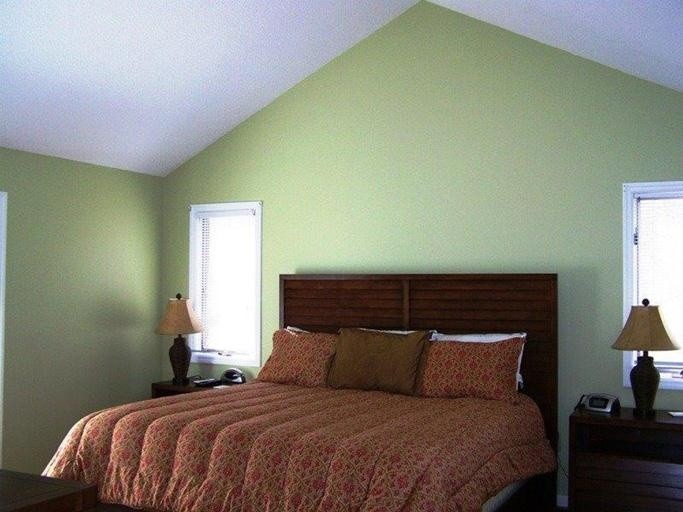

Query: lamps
left=151, top=293, right=205, bottom=386
left=611, top=299, right=677, bottom=415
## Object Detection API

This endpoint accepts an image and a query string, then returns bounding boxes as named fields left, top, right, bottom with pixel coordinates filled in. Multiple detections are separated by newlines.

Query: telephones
left=574, top=393, right=620, bottom=413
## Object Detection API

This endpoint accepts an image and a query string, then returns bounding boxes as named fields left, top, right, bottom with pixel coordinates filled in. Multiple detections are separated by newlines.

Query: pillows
left=255, top=329, right=337, bottom=388
left=285, top=325, right=419, bottom=336
left=326, top=329, right=429, bottom=395
left=431, top=331, right=526, bottom=391
left=411, top=334, right=524, bottom=406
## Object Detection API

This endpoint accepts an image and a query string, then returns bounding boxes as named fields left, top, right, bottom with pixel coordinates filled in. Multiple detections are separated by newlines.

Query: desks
left=0, top=470, right=97, bottom=512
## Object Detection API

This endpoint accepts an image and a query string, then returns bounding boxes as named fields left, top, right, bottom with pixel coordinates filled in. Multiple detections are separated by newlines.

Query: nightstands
left=150, top=377, right=211, bottom=398
left=566, top=404, right=682, bottom=512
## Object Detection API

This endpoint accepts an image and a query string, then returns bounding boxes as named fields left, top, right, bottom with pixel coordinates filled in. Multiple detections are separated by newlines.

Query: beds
left=42, top=271, right=561, bottom=512
